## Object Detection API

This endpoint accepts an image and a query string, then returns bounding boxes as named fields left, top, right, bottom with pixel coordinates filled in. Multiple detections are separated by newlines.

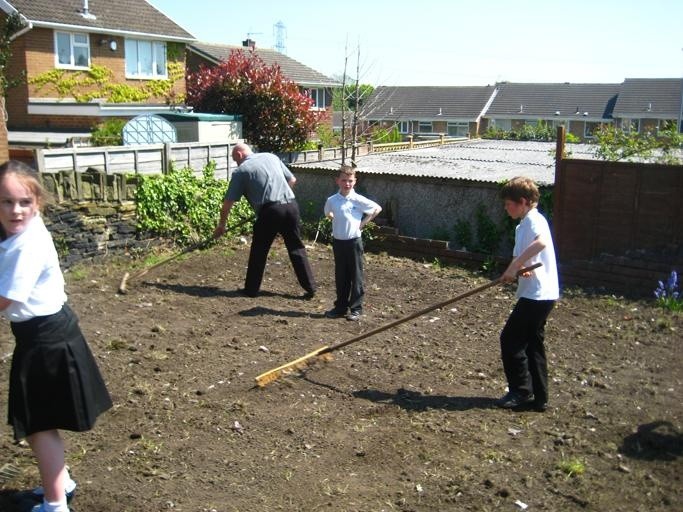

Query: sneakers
left=325, top=311, right=360, bottom=321
left=496, top=392, right=548, bottom=412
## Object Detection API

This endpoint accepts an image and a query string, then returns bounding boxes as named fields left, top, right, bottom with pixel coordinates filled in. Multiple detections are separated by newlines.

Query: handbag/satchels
left=617, top=421, right=683, bottom=461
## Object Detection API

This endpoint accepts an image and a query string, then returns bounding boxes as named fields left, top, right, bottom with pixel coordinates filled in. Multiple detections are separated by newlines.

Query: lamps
left=94, top=37, right=119, bottom=52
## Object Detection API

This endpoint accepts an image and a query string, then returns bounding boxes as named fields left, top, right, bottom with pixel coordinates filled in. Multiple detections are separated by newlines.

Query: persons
left=324, top=166, right=382, bottom=322
left=500, top=176, right=559, bottom=411
left=213, top=143, right=318, bottom=298
left=0, top=159, right=113, bottom=512
